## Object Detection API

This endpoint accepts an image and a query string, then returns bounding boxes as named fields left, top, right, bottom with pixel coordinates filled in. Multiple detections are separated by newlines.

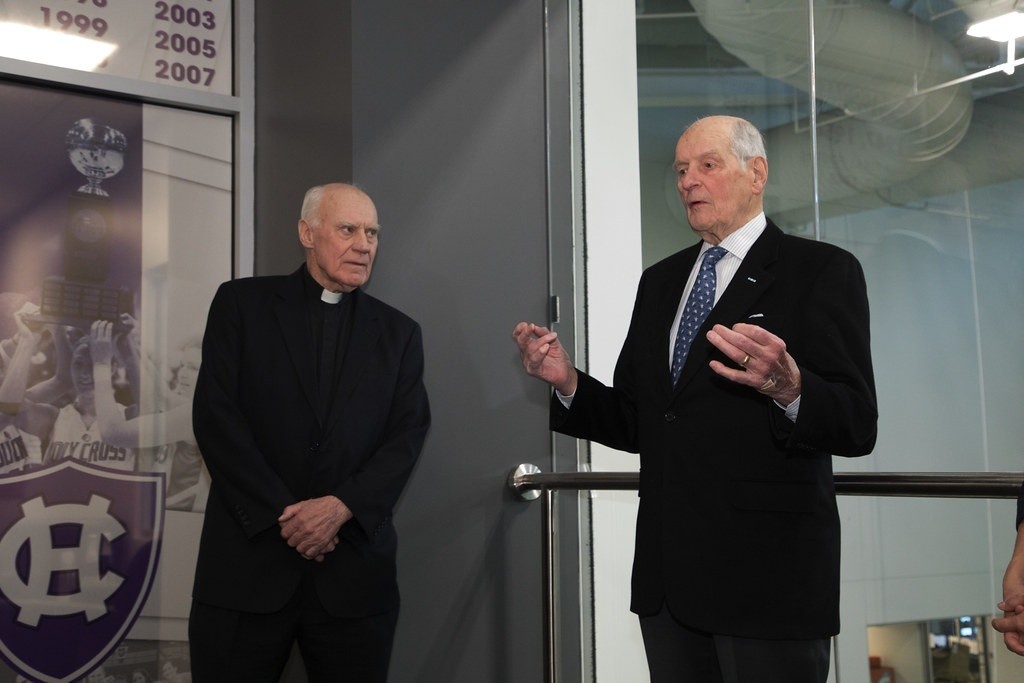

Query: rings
left=740, top=355, right=750, bottom=366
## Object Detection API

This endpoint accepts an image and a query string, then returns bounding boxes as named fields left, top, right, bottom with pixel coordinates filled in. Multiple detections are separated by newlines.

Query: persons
left=187, top=182, right=433, bottom=682
left=512, top=114, right=877, bottom=682
left=0, top=302, right=204, bottom=511
left=992, top=480, right=1024, bottom=657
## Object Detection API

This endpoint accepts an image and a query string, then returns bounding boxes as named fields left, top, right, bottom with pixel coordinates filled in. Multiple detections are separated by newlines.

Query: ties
left=671, top=246, right=728, bottom=385
